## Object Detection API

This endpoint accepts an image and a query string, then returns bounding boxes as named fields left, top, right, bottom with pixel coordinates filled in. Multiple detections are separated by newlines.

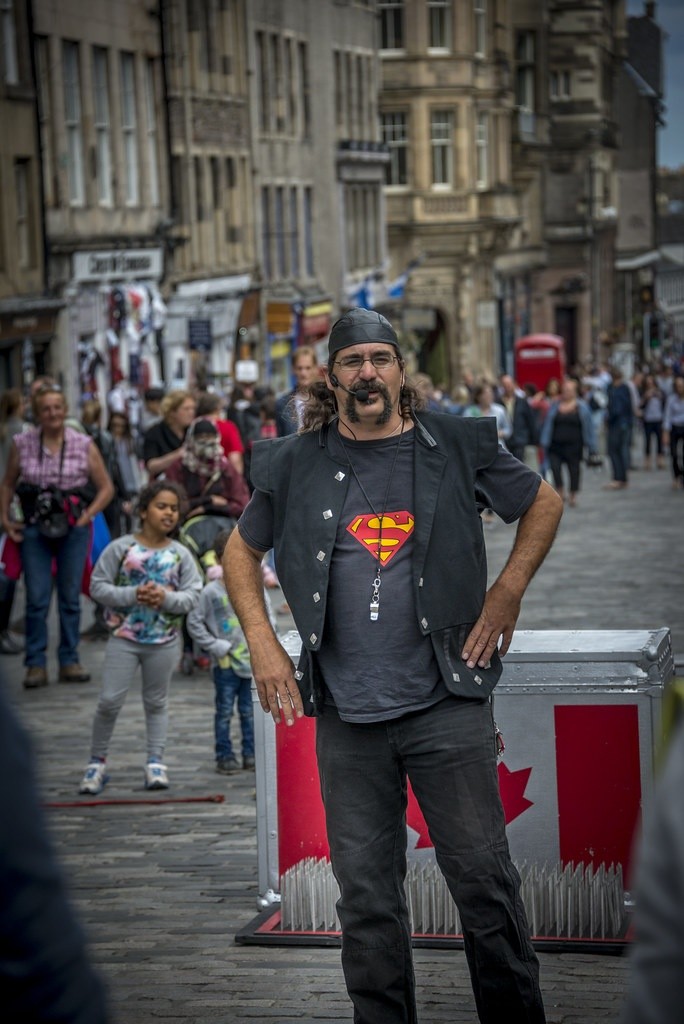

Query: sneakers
left=77, top=758, right=109, bottom=795
left=143, top=758, right=170, bottom=792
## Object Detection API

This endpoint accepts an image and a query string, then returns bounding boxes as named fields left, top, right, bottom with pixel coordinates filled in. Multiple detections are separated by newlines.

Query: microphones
left=337, top=382, right=369, bottom=402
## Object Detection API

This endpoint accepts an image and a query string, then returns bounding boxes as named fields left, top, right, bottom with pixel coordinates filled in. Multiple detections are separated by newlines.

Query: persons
left=82, top=378, right=276, bottom=676
left=274, top=346, right=324, bottom=437
left=623, top=736, right=684, bottom=1024
left=1, top=388, right=35, bottom=655
left=186, top=531, right=274, bottom=775
left=80, top=482, right=204, bottom=794
left=220, top=307, right=563, bottom=1024
left=0, top=383, right=115, bottom=689
left=412, top=364, right=684, bottom=524
left=0, top=675, right=109, bottom=1023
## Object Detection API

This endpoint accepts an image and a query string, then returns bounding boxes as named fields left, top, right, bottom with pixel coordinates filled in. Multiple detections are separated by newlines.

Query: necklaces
left=336, top=420, right=405, bottom=622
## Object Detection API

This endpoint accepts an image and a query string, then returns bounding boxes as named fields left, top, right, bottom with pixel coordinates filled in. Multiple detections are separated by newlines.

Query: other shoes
left=58, top=662, right=90, bottom=683
left=607, top=479, right=629, bottom=490
left=214, top=757, right=241, bottom=775
left=0, top=633, right=25, bottom=655
left=80, top=623, right=109, bottom=643
left=569, top=494, right=577, bottom=505
left=242, top=756, right=255, bottom=772
left=22, top=665, right=49, bottom=689
left=181, top=653, right=195, bottom=676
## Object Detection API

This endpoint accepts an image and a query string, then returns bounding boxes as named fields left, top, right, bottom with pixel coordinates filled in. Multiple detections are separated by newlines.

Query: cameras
left=32, top=484, right=64, bottom=520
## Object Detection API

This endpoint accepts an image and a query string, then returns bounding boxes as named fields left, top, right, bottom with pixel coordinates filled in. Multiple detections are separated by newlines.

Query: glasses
left=332, top=354, right=399, bottom=371
left=33, top=384, right=63, bottom=401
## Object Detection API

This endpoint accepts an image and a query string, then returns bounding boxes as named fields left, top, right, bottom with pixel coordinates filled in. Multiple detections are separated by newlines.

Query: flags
left=351, top=276, right=375, bottom=309
left=390, top=269, right=408, bottom=297
